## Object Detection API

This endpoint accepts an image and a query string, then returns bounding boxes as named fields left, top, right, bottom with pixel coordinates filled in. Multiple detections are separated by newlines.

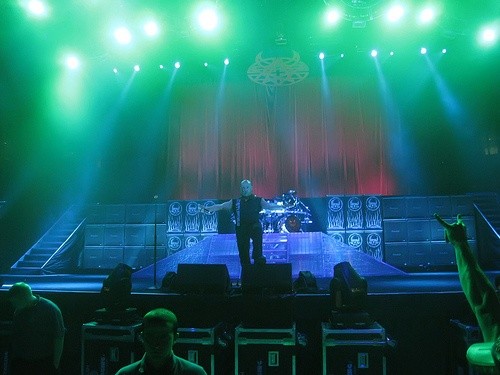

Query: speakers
left=240, top=263, right=292, bottom=294
left=175, top=263, right=233, bottom=295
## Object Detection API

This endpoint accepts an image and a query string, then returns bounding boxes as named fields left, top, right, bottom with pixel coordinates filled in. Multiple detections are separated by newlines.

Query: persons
left=7, top=283, right=67, bottom=375
left=434, top=214, right=500, bottom=343
left=200, top=180, right=295, bottom=266
left=114, top=309, right=208, bottom=375
